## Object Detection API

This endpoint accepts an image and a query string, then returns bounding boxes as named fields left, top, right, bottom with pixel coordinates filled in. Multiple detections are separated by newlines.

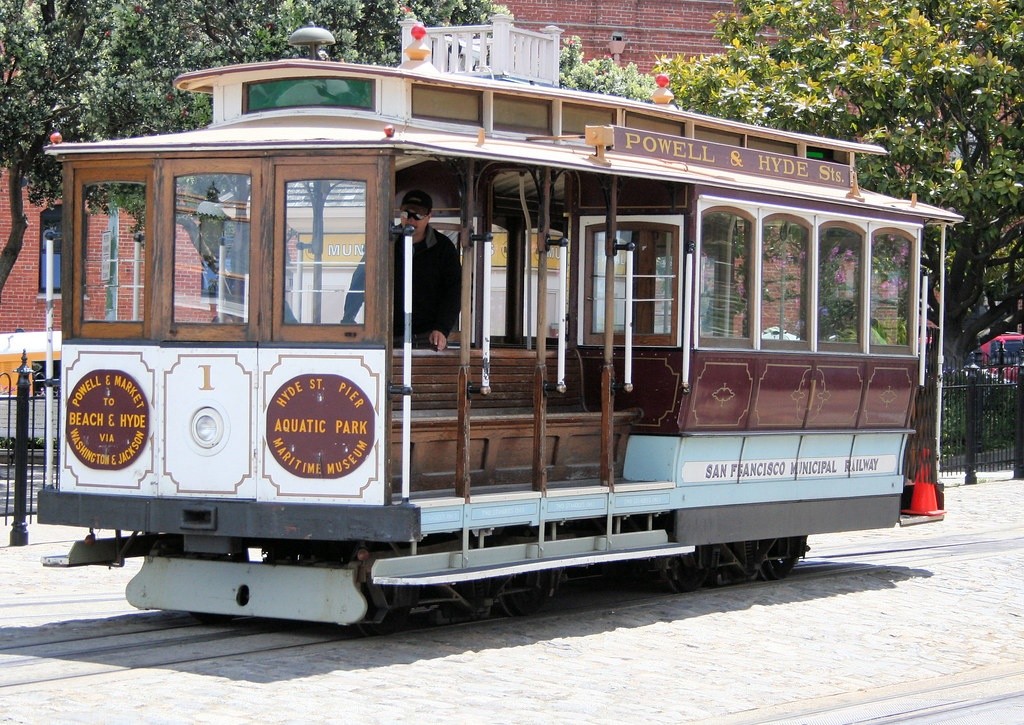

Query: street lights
left=191, top=180, right=231, bottom=324
left=275, top=22, right=353, bottom=324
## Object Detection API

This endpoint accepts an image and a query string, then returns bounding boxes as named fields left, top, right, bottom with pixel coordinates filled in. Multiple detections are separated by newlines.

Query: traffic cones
left=901, top=447, right=948, bottom=516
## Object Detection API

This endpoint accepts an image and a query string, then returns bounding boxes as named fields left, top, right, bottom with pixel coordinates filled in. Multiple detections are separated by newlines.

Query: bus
left=34, top=61, right=965, bottom=638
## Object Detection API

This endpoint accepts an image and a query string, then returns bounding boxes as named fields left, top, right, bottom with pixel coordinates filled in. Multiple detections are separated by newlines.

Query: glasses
left=402, top=209, right=428, bottom=221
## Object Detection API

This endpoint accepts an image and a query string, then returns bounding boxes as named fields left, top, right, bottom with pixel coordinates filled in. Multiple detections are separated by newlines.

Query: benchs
left=392, top=349, right=644, bottom=492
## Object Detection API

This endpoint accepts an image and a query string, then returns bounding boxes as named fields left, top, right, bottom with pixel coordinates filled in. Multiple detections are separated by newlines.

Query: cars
left=976, top=333, right=1024, bottom=382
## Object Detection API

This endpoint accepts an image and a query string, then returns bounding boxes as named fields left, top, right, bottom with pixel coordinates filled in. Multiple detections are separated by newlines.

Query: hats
left=402, top=190, right=432, bottom=208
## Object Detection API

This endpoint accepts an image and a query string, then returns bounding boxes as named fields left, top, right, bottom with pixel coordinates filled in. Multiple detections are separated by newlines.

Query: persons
left=340, top=189, right=462, bottom=351
